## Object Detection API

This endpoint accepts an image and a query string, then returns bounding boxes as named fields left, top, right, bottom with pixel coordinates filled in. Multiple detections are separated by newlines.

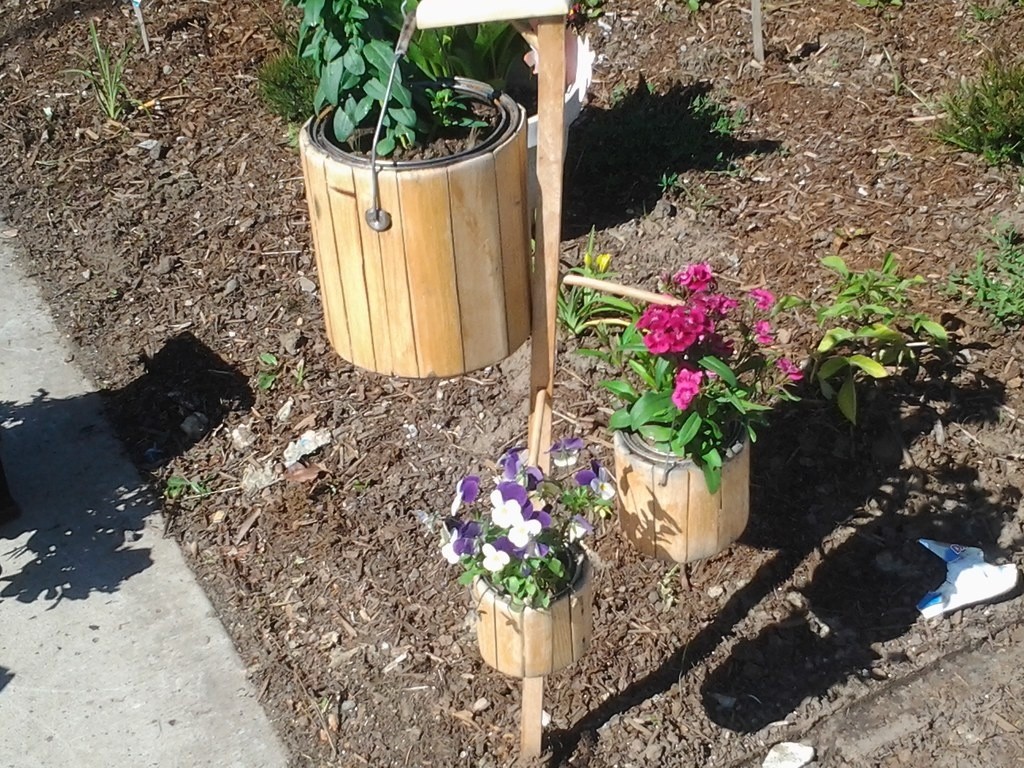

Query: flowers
left=573, top=265, right=803, bottom=494
left=442, top=434, right=619, bottom=614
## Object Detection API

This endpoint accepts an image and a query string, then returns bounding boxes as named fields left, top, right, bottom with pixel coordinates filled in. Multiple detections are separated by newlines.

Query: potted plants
left=278, top=0, right=532, bottom=379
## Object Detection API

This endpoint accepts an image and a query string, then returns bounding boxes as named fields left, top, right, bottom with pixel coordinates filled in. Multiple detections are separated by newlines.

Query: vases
left=615, top=429, right=749, bottom=565
left=473, top=541, right=592, bottom=679
